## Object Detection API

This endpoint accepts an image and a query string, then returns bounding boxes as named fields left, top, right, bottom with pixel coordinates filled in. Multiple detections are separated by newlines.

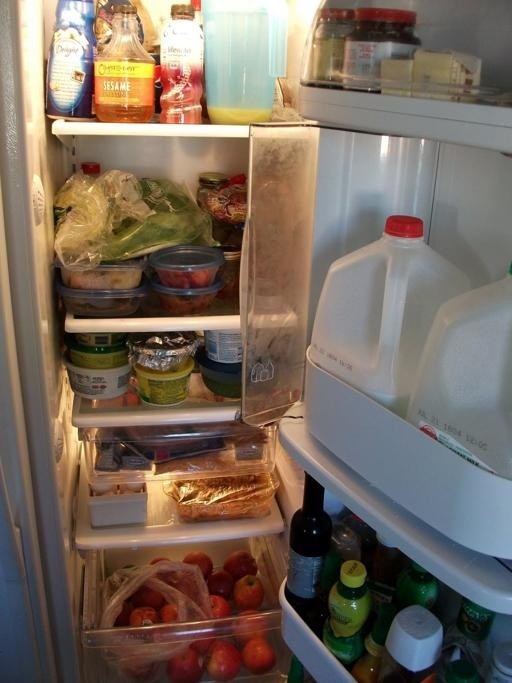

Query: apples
left=112, top=551, right=276, bottom=683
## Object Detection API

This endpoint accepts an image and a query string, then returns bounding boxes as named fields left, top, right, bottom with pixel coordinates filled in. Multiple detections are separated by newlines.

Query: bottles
left=46, top=0, right=287, bottom=125
left=80, top=162, right=101, bottom=179
left=286, top=469, right=512, bottom=683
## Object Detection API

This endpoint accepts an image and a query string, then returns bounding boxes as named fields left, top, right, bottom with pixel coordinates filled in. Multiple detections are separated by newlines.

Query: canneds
left=341, top=6, right=423, bottom=94
left=196, top=171, right=228, bottom=208
left=485, top=640, right=512, bottom=683
left=311, top=7, right=355, bottom=82
left=214, top=245, right=241, bottom=300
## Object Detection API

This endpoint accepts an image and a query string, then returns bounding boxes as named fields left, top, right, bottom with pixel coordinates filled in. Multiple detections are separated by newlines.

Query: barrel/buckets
left=403, top=258, right=511, bottom=483
left=306, top=213, right=470, bottom=422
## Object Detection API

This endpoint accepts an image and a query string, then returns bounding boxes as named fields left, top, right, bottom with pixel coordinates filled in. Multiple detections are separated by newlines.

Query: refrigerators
left=0, top=0, right=512, bottom=682
